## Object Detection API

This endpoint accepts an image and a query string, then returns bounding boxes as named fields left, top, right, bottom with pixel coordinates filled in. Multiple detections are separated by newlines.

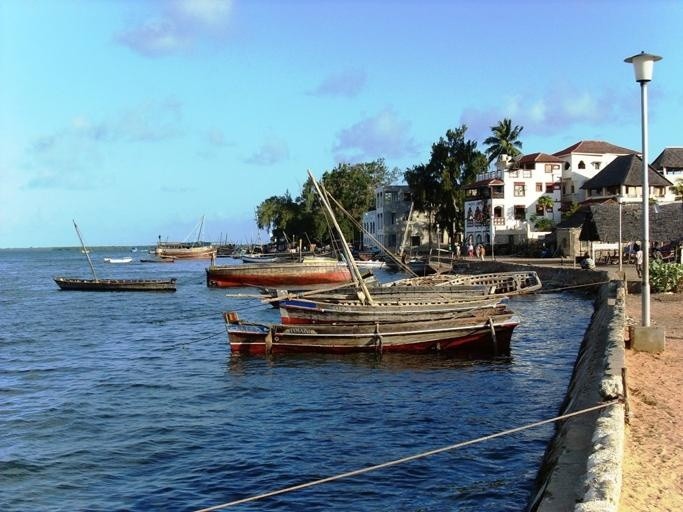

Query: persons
left=579, top=254, right=595, bottom=269
left=634, top=246, right=642, bottom=278
left=454, top=241, right=485, bottom=260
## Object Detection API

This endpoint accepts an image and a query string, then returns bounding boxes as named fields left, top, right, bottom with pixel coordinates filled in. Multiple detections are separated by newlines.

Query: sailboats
left=156, top=212, right=352, bottom=292
left=55, top=218, right=177, bottom=291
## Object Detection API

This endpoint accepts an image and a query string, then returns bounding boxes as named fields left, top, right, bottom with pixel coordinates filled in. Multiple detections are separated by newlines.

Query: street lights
left=617, top=195, right=624, bottom=270
left=624, top=52, right=662, bottom=329
